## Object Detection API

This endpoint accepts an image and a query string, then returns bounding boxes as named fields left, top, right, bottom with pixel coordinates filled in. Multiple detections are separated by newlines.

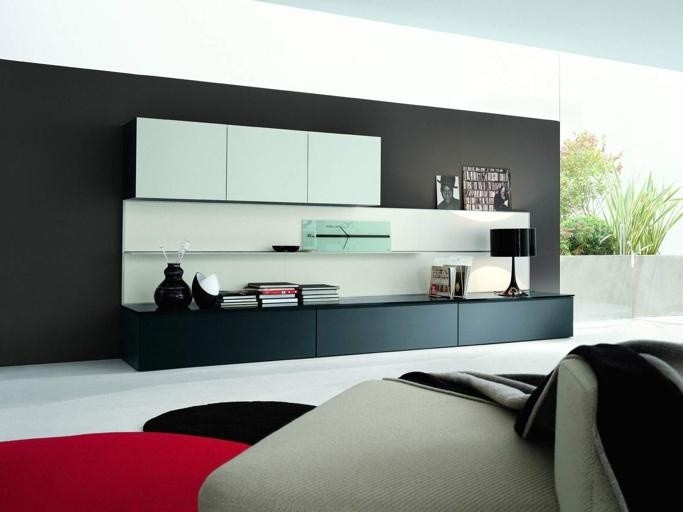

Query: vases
left=153, top=263, right=191, bottom=308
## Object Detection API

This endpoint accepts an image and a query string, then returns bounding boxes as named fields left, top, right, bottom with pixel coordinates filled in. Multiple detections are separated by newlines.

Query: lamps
left=489, top=229, right=536, bottom=298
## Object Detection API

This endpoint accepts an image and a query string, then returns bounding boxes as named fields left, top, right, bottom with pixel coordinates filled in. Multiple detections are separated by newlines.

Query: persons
left=494, top=186, right=509, bottom=211
left=436, top=175, right=459, bottom=209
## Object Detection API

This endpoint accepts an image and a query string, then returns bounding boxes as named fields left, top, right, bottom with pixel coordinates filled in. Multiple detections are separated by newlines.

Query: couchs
left=0, top=430, right=247, bottom=512
left=142, top=400, right=318, bottom=450
left=196, top=376, right=683, bottom=512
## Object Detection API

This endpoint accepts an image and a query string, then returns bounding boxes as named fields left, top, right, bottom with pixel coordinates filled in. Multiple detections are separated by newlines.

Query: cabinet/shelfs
left=114, top=117, right=574, bottom=372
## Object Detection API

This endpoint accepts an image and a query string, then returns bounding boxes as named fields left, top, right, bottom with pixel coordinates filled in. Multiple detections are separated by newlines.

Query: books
left=430, top=266, right=455, bottom=298
left=216, top=282, right=340, bottom=308
left=443, top=264, right=469, bottom=297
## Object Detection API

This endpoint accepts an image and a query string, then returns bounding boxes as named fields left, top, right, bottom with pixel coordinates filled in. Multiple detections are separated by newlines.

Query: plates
left=272, top=245, right=299, bottom=251
